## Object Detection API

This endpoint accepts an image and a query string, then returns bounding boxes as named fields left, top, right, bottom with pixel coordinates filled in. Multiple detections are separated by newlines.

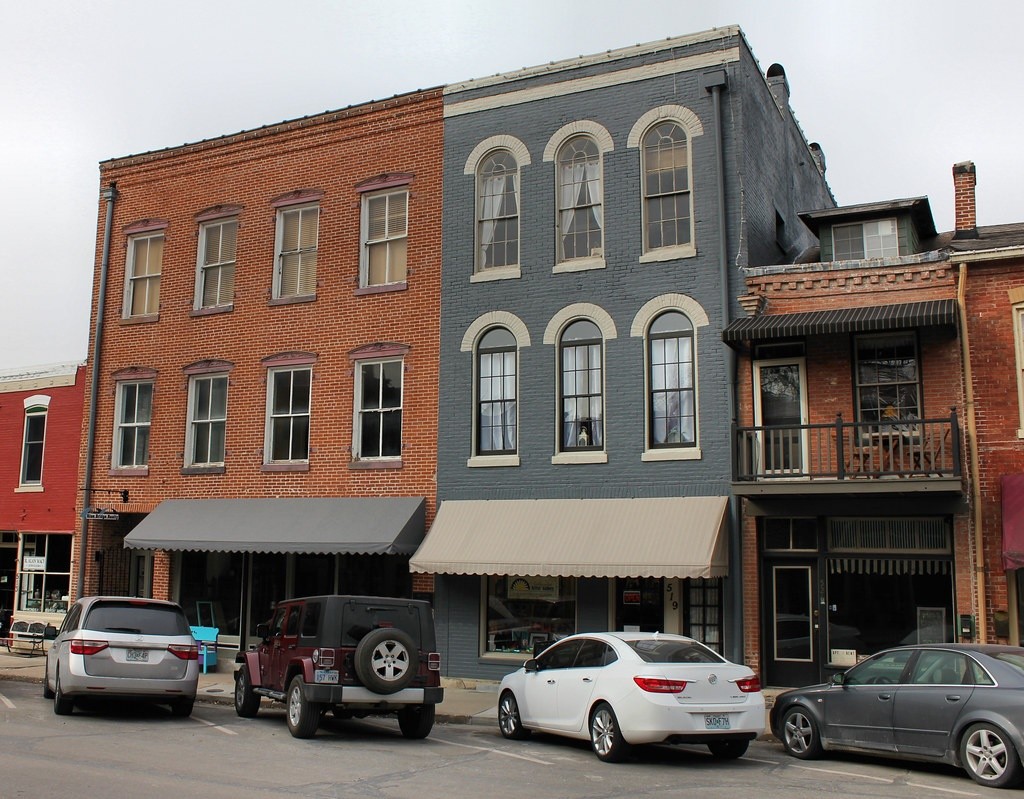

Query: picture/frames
left=196, top=600, right=215, bottom=628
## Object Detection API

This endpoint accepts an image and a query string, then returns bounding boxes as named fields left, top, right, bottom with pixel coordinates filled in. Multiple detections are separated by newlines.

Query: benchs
left=189, top=625, right=220, bottom=675
left=0, top=620, right=48, bottom=658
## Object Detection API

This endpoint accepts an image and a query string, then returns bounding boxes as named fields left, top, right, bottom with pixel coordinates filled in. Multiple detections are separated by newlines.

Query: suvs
left=234, top=595, right=446, bottom=740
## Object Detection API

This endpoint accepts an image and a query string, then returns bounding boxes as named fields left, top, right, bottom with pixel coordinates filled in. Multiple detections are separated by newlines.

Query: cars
left=768, top=642, right=1024, bottom=788
left=496, top=630, right=766, bottom=764
left=43, top=596, right=201, bottom=718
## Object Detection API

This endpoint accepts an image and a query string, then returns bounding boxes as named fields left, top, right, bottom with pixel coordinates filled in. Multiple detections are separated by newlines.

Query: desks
left=862, top=431, right=920, bottom=480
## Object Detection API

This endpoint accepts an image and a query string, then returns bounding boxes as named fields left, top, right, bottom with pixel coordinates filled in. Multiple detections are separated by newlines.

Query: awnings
left=408, top=496, right=730, bottom=579
left=1000, top=472, right=1024, bottom=571
left=123, top=497, right=427, bottom=556
left=722, top=299, right=957, bottom=353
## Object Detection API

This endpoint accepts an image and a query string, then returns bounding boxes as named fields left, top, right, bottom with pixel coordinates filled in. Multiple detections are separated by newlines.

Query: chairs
left=906, top=428, right=950, bottom=478
left=831, top=435, right=870, bottom=478
